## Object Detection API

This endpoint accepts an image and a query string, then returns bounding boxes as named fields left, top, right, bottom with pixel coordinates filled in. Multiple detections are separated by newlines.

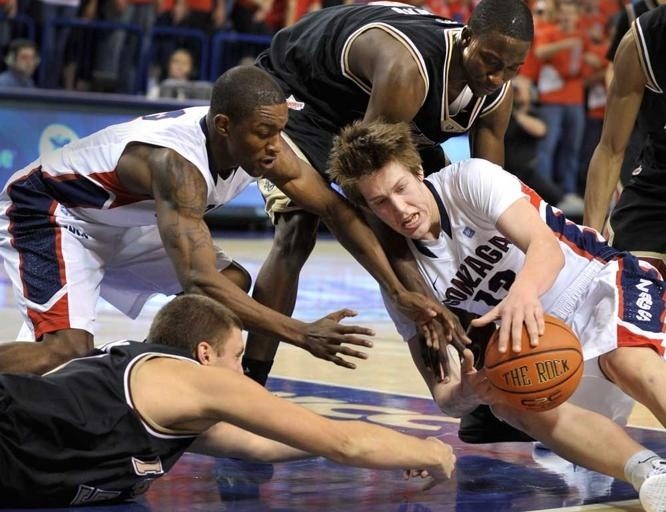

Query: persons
left=324, top=117, right=666, bottom=512
left=0, top=292, right=457, bottom=511
left=1, top=1, right=323, bottom=94
left=0, top=62, right=472, bottom=381
left=580, top=0, right=666, bottom=278
left=415, top=1, right=620, bottom=215
left=231, top=0, right=534, bottom=384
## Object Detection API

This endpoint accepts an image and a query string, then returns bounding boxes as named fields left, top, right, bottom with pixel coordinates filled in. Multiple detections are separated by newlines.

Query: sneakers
left=458, top=404, right=537, bottom=443
left=632, top=456, right=666, bottom=512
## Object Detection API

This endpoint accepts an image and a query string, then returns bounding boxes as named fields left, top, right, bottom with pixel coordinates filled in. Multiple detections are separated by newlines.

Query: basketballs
left=483, top=313, right=583, bottom=411
